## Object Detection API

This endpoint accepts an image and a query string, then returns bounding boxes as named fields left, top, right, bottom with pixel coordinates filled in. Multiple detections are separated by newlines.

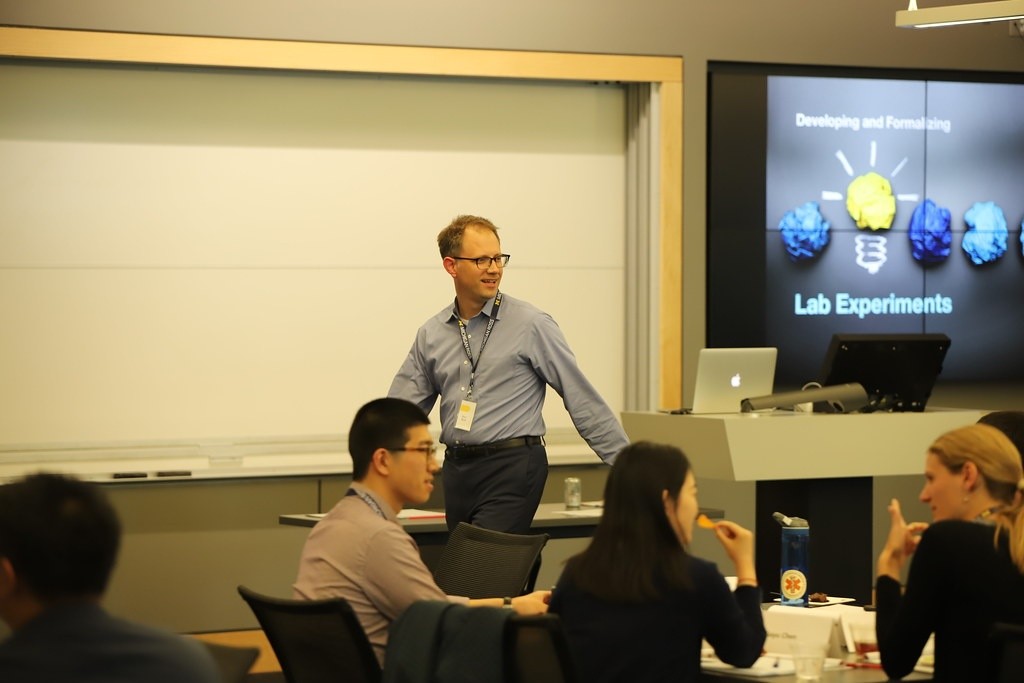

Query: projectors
left=1008, top=19, right=1024, bottom=37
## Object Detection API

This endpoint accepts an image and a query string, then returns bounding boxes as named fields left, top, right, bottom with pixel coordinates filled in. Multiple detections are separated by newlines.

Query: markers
left=156, top=472, right=191, bottom=476
left=114, top=474, right=148, bottom=478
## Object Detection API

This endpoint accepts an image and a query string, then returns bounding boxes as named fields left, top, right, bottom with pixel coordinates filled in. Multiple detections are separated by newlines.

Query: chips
left=697, top=514, right=715, bottom=528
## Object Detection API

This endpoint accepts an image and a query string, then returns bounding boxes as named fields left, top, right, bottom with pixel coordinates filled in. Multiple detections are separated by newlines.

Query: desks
left=280, top=501, right=724, bottom=546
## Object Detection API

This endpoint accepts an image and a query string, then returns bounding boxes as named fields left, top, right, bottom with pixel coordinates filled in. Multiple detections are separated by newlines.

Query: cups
left=791, top=636, right=831, bottom=680
left=780, top=526, right=809, bottom=608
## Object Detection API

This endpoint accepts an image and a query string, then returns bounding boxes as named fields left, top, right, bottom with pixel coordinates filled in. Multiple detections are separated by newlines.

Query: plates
left=774, top=597, right=855, bottom=606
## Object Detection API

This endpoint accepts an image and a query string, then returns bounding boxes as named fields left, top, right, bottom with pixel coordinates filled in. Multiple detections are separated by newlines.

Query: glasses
left=388, top=444, right=439, bottom=458
left=453, top=253, right=511, bottom=270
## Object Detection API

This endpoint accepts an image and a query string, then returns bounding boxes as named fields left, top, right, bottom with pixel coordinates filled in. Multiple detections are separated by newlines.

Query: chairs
left=433, top=522, right=551, bottom=599
left=239, top=586, right=383, bottom=683
left=383, top=600, right=581, bottom=683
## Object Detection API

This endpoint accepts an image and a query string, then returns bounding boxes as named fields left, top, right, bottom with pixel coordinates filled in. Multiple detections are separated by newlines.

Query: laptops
left=659, top=348, right=778, bottom=414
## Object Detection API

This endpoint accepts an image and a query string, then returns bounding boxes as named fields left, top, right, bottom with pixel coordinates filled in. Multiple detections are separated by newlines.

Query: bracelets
left=736, top=577, right=757, bottom=587
left=502, top=597, right=512, bottom=610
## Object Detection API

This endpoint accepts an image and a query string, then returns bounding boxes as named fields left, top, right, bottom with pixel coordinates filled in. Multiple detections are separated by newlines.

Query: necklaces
left=344, top=487, right=387, bottom=520
left=972, top=504, right=1007, bottom=519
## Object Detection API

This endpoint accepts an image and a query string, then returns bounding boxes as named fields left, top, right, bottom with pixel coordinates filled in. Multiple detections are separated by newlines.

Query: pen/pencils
left=841, top=662, right=880, bottom=668
left=774, top=657, right=780, bottom=667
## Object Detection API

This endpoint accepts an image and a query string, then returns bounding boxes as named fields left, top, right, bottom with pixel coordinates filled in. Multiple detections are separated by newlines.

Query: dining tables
left=700, top=600, right=935, bottom=683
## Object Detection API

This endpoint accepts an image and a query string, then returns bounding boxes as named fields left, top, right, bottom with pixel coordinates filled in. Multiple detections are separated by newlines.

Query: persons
left=876, top=411, right=1024, bottom=683
left=810, top=584, right=829, bottom=602
left=547, top=440, right=767, bottom=683
left=291, top=397, right=553, bottom=671
left=387, top=215, right=630, bottom=535
left=0, top=472, right=225, bottom=683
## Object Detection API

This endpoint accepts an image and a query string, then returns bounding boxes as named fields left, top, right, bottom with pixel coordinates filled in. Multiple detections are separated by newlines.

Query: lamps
left=896, top=0, right=1024, bottom=31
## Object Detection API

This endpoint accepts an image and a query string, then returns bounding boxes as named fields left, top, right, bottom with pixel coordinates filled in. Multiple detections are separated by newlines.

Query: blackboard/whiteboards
left=0, top=24, right=683, bottom=483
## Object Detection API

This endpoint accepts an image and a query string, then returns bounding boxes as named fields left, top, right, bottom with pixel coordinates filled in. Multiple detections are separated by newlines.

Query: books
left=555, top=501, right=603, bottom=518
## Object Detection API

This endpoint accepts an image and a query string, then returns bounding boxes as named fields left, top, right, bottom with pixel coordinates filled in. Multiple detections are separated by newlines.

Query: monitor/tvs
left=812, top=333, right=950, bottom=413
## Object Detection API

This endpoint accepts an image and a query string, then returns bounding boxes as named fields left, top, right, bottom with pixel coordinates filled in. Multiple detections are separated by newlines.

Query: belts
left=445, top=435, right=542, bottom=461
